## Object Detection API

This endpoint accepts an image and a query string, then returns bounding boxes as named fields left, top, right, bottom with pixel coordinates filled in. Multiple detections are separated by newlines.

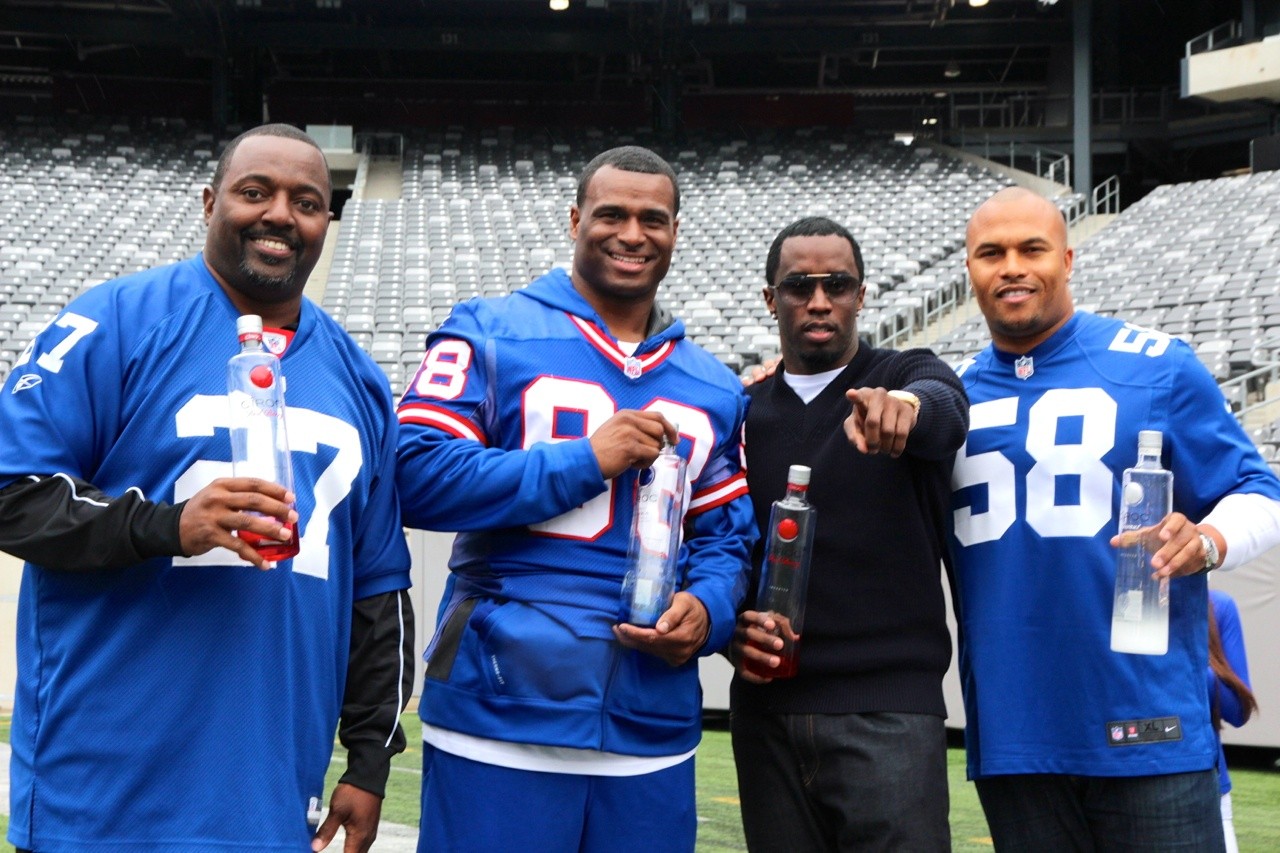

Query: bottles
left=226, top=315, right=300, bottom=563
left=742, top=464, right=817, bottom=678
left=619, top=422, right=688, bottom=630
left=1109, top=430, right=1174, bottom=656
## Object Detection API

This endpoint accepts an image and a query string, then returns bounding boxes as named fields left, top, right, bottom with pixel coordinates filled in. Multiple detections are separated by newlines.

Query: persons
left=741, top=188, right=1280, bottom=853
left=0, top=123, right=415, bottom=853
left=396, top=145, right=758, bottom=853
left=716, top=217, right=971, bottom=853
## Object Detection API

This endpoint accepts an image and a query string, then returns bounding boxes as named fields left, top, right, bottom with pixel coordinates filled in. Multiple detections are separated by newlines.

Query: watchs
left=1197, top=533, right=1219, bottom=576
left=887, top=390, right=921, bottom=416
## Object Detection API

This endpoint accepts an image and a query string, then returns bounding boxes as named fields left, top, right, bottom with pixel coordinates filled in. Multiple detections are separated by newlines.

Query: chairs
left=0, top=109, right=1280, bottom=473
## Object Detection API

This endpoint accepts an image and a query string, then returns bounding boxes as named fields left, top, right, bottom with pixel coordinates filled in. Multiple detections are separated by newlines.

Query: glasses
left=769, top=272, right=862, bottom=306
